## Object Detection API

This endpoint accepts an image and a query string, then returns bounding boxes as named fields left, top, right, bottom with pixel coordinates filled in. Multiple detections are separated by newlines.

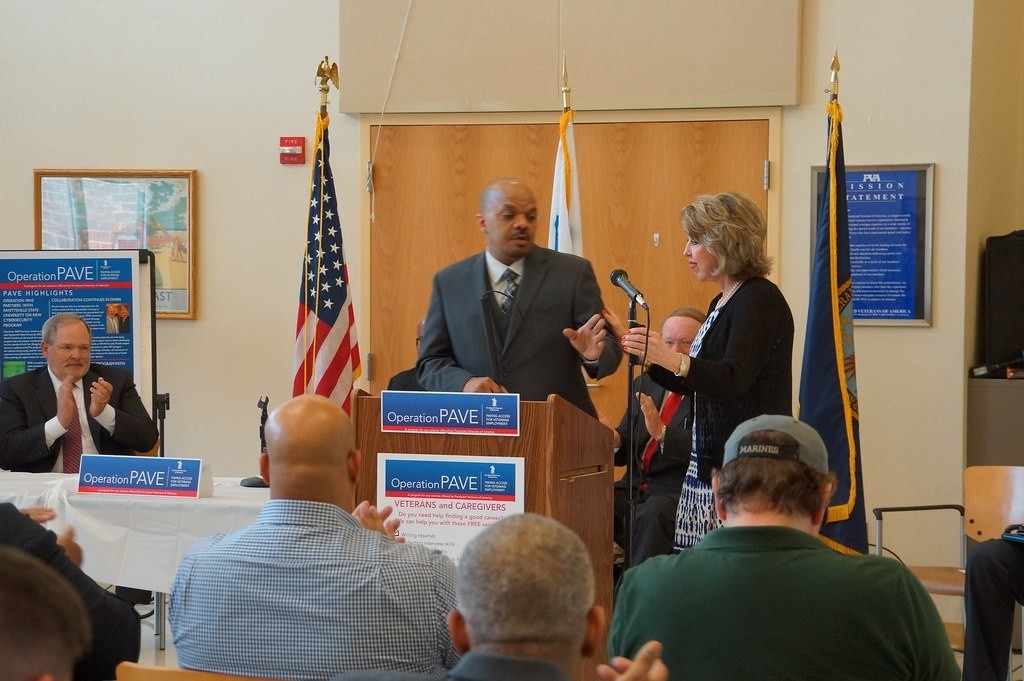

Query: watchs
left=674, top=353, right=686, bottom=377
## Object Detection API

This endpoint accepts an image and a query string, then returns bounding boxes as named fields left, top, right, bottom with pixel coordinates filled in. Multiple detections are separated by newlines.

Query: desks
left=0, top=469, right=269, bottom=650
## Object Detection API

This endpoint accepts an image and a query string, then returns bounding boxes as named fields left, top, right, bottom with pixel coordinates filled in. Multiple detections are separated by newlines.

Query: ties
left=62, top=395, right=83, bottom=474
left=643, top=392, right=683, bottom=473
left=501, top=270, right=519, bottom=313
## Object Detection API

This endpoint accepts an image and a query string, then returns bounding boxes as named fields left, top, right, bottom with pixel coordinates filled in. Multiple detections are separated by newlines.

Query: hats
left=722, top=414, right=829, bottom=473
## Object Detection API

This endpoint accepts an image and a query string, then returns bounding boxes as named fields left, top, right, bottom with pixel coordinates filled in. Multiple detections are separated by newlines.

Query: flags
left=547, top=113, right=584, bottom=256
left=797, top=101, right=868, bottom=556
left=292, top=112, right=362, bottom=418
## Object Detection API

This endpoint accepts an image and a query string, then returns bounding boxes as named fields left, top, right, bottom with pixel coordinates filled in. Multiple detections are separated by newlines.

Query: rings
left=99, top=399, right=106, bottom=402
left=639, top=350, right=644, bottom=359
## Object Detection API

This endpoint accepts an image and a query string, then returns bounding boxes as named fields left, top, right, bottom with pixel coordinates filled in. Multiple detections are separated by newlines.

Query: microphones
left=611, top=269, right=648, bottom=310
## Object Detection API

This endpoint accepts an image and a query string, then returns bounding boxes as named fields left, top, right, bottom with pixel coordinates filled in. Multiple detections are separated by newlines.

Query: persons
left=330, top=514, right=668, bottom=681
left=106, top=305, right=130, bottom=333
left=962, top=539, right=1024, bottom=681
left=0, top=502, right=141, bottom=681
left=620, top=192, right=794, bottom=554
left=167, top=393, right=463, bottom=681
left=0, top=313, right=159, bottom=620
left=0, top=547, right=91, bottom=681
left=597, top=306, right=703, bottom=588
left=387, top=319, right=425, bottom=391
left=416, top=179, right=624, bottom=419
left=607, top=415, right=961, bottom=681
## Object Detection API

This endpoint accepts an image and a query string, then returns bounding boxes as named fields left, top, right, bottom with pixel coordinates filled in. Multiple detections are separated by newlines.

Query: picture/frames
left=31, top=170, right=196, bottom=320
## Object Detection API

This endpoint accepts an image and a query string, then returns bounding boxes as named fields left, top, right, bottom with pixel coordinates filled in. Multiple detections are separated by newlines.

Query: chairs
left=963, top=465, right=1024, bottom=544
